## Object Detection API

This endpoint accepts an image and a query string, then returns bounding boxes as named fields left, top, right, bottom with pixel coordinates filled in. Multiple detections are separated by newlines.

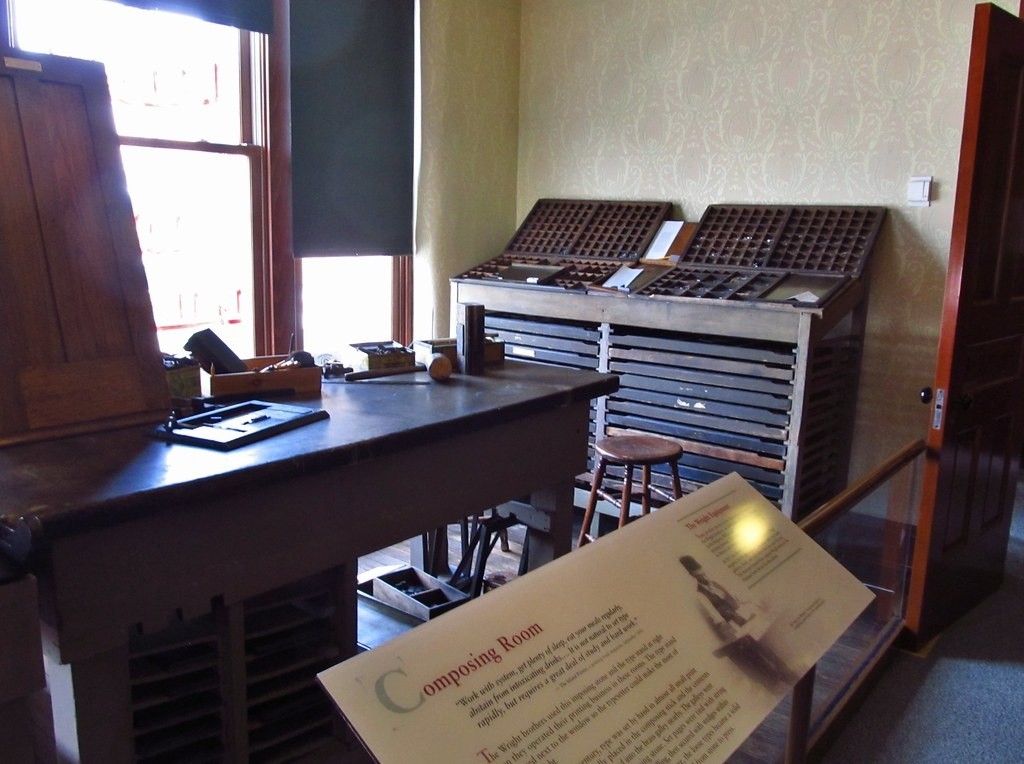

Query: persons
left=677, top=555, right=740, bottom=629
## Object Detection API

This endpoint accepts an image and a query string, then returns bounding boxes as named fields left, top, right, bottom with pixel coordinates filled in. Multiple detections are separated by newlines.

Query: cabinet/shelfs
left=0, top=46, right=169, bottom=445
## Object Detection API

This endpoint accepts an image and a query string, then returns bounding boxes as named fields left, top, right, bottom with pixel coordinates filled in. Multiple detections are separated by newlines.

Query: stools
left=579, top=433, right=684, bottom=551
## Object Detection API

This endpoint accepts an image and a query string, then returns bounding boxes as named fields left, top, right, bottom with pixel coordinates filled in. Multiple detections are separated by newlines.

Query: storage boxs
left=371, top=566, right=474, bottom=620
left=349, top=338, right=417, bottom=371
left=483, top=336, right=509, bottom=362
left=357, top=578, right=424, bottom=653
left=417, top=335, right=457, bottom=370
left=165, top=351, right=204, bottom=404
left=208, top=352, right=327, bottom=396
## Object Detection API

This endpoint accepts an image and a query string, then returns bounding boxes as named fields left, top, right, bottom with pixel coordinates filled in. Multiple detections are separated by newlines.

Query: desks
left=0, top=360, right=620, bottom=764
